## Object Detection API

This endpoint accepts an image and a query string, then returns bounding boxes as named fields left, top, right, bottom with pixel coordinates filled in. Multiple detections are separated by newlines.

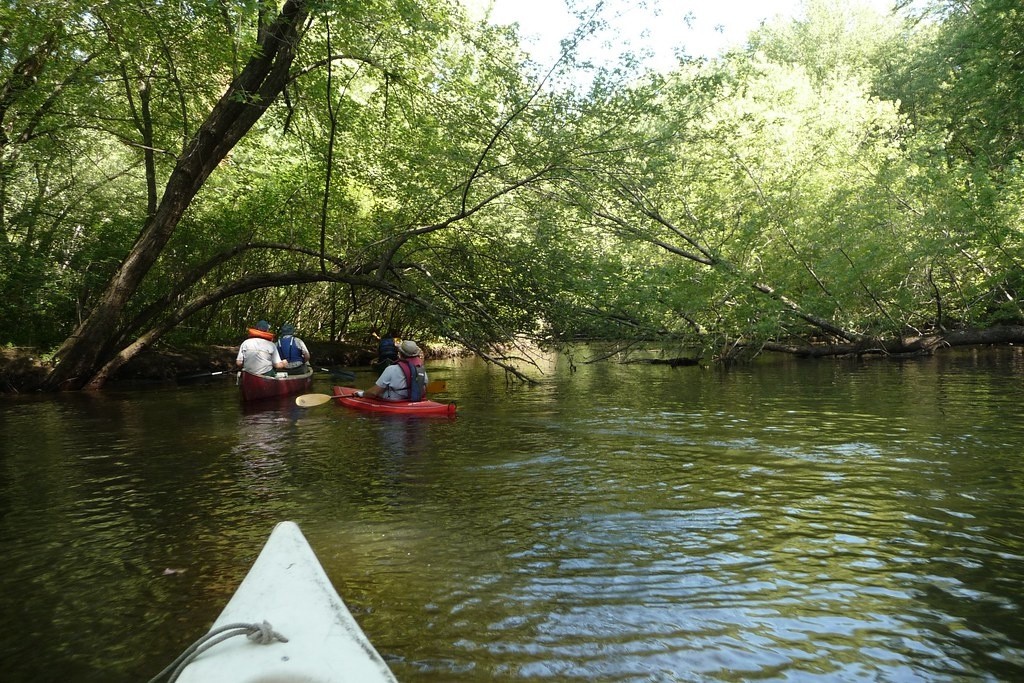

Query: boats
left=238, top=361, right=314, bottom=402
left=329, top=383, right=456, bottom=415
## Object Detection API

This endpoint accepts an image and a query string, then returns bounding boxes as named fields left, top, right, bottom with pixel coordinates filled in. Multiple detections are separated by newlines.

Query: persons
left=273, top=325, right=311, bottom=375
left=355, top=340, right=429, bottom=401
left=235, top=320, right=288, bottom=377
left=376, top=330, right=403, bottom=363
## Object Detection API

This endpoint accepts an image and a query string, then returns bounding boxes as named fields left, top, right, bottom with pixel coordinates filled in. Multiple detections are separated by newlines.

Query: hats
left=257, top=320, right=269, bottom=331
left=398, top=340, right=421, bottom=356
left=282, top=325, right=294, bottom=335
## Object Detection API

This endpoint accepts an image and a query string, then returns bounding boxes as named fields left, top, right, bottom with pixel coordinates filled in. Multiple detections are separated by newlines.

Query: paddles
left=296, top=382, right=448, bottom=408
left=176, top=366, right=241, bottom=380
left=305, top=363, right=356, bottom=381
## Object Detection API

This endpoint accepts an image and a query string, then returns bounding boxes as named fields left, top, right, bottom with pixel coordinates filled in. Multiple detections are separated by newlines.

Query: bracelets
left=357, top=391, right=364, bottom=398
